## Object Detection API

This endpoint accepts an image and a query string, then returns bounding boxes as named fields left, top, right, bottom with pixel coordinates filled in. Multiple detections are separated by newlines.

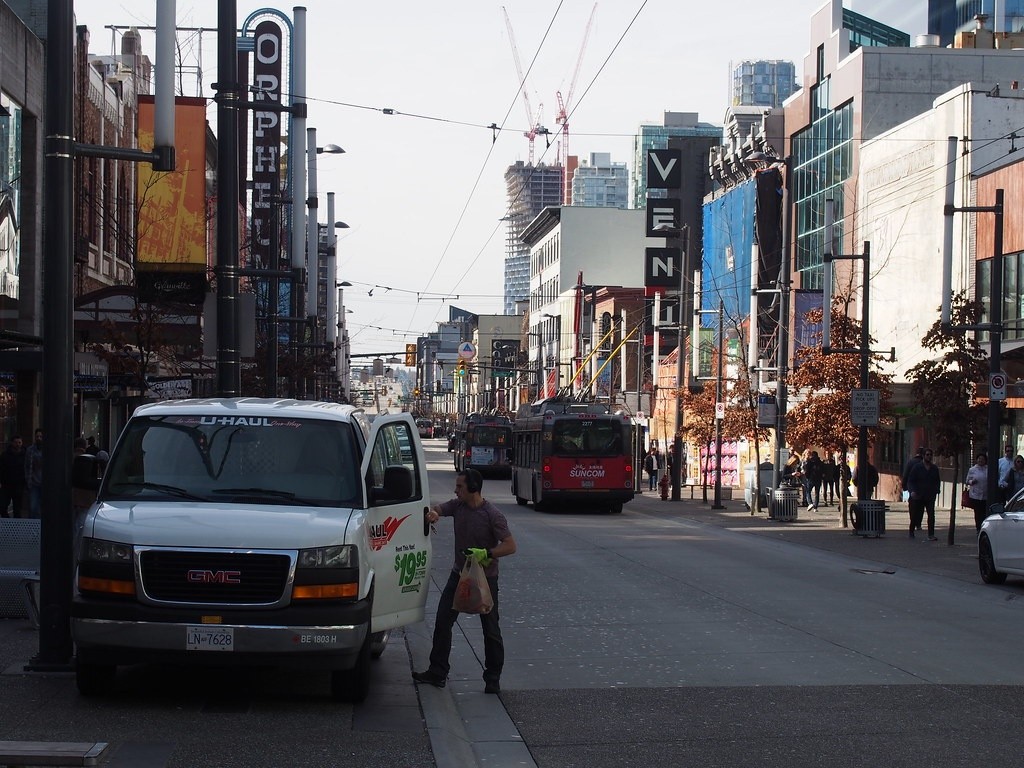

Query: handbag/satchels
left=902, top=490, right=909, bottom=502
left=451, top=554, right=494, bottom=615
left=799, top=473, right=808, bottom=483
left=961, top=479, right=976, bottom=509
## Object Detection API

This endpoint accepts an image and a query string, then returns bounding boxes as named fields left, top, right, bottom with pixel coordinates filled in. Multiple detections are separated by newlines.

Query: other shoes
left=927, top=536, right=937, bottom=540
left=909, top=532, right=915, bottom=538
left=917, top=526, right=922, bottom=530
left=807, top=504, right=814, bottom=511
left=814, top=509, right=818, bottom=512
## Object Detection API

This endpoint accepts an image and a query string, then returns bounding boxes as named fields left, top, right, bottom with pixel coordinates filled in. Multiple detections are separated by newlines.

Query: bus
left=454, top=414, right=510, bottom=479
left=511, top=397, right=635, bottom=514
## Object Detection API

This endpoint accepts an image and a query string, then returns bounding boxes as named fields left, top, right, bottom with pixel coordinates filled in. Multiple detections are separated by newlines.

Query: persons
left=804, top=451, right=824, bottom=511
left=74, top=447, right=85, bottom=456
left=0, top=434, right=28, bottom=518
left=97, top=451, right=109, bottom=463
left=85, top=436, right=101, bottom=456
left=908, top=447, right=940, bottom=541
left=74, top=437, right=86, bottom=448
left=832, top=455, right=852, bottom=512
left=965, top=453, right=988, bottom=534
left=641, top=445, right=674, bottom=490
left=783, top=454, right=801, bottom=483
left=901, top=448, right=924, bottom=530
left=410, top=469, right=516, bottom=692
left=999, top=446, right=1024, bottom=511
left=852, top=454, right=879, bottom=500
left=800, top=448, right=812, bottom=506
left=25, top=428, right=42, bottom=519
left=822, top=458, right=836, bottom=506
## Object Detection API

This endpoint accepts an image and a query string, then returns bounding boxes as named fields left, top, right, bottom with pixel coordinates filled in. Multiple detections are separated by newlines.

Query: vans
left=71, top=398, right=434, bottom=706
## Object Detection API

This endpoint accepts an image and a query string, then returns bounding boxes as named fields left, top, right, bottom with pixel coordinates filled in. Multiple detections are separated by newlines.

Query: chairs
left=283, top=430, right=352, bottom=501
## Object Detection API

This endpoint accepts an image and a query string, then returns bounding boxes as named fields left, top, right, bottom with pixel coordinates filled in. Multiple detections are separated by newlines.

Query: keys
left=431, top=525, right=438, bottom=535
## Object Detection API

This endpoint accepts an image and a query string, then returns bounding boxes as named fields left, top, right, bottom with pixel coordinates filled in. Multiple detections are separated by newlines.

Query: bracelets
left=487, top=548, right=492, bottom=558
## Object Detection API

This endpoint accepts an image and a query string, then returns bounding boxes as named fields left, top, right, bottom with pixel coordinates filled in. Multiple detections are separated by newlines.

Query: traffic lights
left=458, top=358, right=468, bottom=377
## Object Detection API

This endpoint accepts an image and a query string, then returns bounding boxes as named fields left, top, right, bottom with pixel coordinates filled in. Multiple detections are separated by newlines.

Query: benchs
left=0, top=517, right=41, bottom=630
left=681, top=484, right=712, bottom=499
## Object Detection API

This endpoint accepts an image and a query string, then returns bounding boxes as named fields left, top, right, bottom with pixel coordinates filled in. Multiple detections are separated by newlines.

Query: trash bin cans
left=857, top=500, right=886, bottom=535
left=774, top=489, right=799, bottom=520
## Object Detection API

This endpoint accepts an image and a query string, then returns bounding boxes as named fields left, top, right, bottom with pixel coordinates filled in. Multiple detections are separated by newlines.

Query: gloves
left=462, top=547, right=494, bottom=567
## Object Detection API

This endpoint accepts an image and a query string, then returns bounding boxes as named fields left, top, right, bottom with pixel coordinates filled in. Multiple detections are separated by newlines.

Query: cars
left=388, top=436, right=413, bottom=461
left=362, top=398, right=374, bottom=407
left=415, top=418, right=433, bottom=439
left=977, top=487, right=1024, bottom=584
left=448, top=435, right=455, bottom=452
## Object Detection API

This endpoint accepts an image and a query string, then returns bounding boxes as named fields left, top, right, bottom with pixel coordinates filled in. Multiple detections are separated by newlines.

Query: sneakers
left=411, top=669, right=449, bottom=687
left=485, top=679, right=501, bottom=693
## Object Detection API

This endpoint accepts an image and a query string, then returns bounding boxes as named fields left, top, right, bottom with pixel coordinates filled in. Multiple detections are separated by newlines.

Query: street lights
left=525, top=332, right=542, bottom=401
left=422, top=361, right=487, bottom=414
left=502, top=345, right=520, bottom=412
left=540, top=314, right=561, bottom=395
left=267, top=144, right=353, bottom=401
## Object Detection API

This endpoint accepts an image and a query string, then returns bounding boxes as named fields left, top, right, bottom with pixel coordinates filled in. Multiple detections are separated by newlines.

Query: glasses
left=1006, top=450, right=1014, bottom=452
left=1017, top=461, right=1023, bottom=463
